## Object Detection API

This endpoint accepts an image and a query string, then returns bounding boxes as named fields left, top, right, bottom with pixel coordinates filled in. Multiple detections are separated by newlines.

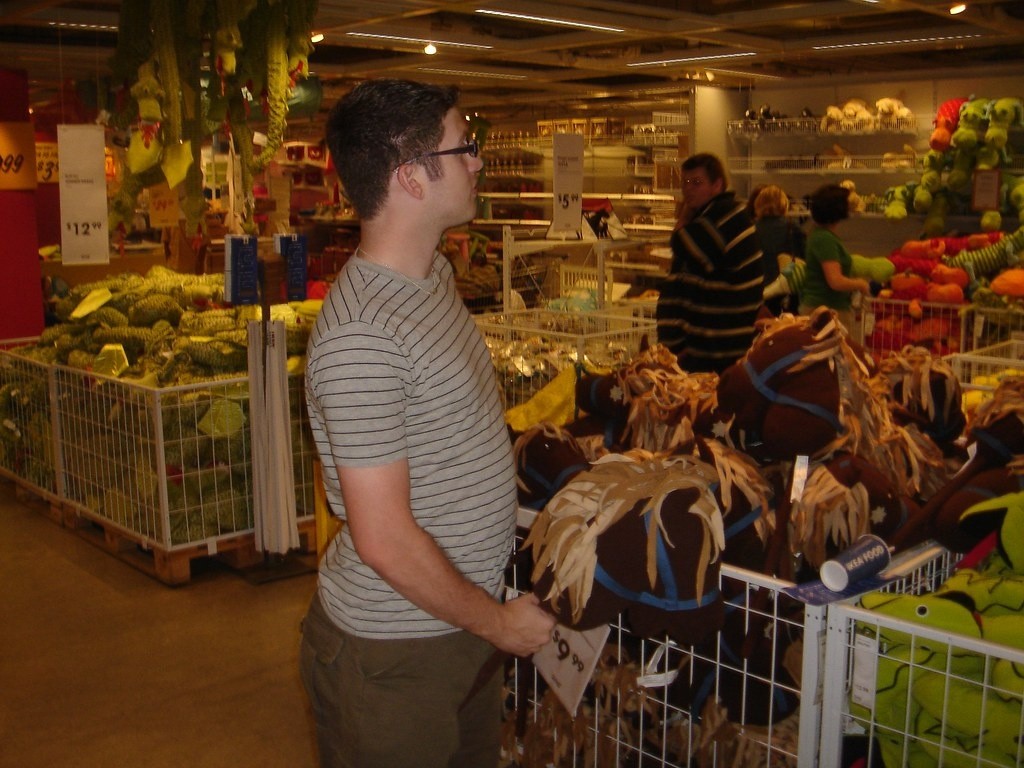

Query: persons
left=299, top=79, right=558, bottom=768
left=805, top=185, right=870, bottom=329
left=656, top=153, right=764, bottom=373
left=746, top=183, right=808, bottom=316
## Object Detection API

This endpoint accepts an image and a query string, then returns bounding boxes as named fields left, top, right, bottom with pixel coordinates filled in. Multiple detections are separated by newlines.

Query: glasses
left=393, top=137, right=480, bottom=174
left=679, top=178, right=712, bottom=186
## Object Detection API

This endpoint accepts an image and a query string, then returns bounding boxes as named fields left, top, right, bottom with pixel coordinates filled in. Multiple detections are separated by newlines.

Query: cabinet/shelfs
left=277, top=82, right=1024, bottom=316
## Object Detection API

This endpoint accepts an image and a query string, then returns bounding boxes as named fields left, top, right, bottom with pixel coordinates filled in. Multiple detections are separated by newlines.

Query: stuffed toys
left=849, top=492, right=1024, bottom=768
left=512, top=306, right=1024, bottom=723
left=885, top=97, right=1024, bottom=238
